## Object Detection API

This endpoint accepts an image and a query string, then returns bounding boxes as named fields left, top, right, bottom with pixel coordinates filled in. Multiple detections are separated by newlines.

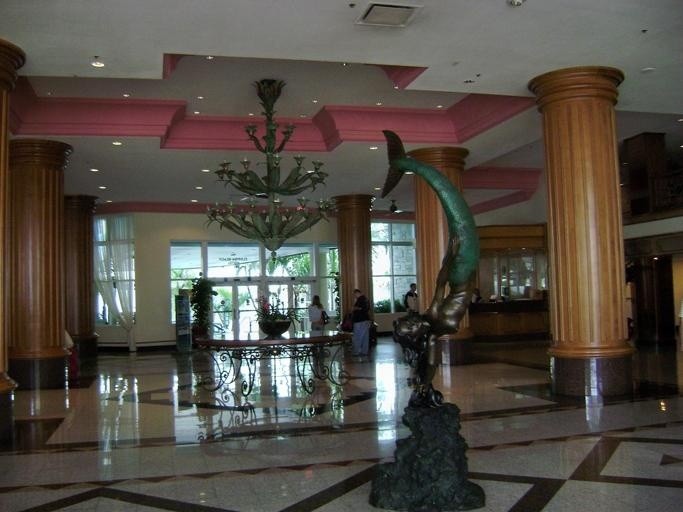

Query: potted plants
left=187, top=271, right=218, bottom=337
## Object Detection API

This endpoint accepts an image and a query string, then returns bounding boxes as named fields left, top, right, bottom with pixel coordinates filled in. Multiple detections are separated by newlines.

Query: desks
left=193, top=330, right=354, bottom=398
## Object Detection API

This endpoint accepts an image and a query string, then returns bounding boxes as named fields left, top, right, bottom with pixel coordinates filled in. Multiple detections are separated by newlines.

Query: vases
left=257, top=322, right=290, bottom=335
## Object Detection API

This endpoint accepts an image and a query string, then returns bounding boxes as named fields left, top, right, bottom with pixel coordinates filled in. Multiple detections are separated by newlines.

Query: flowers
left=253, top=292, right=299, bottom=321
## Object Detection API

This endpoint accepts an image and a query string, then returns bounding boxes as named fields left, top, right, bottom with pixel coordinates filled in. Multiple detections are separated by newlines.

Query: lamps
left=199, top=76, right=338, bottom=262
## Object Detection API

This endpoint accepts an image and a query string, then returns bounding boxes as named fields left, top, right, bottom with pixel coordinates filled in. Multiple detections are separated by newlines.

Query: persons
left=306, top=295, right=330, bottom=355
left=402, top=282, right=418, bottom=317
left=347, top=288, right=372, bottom=358
